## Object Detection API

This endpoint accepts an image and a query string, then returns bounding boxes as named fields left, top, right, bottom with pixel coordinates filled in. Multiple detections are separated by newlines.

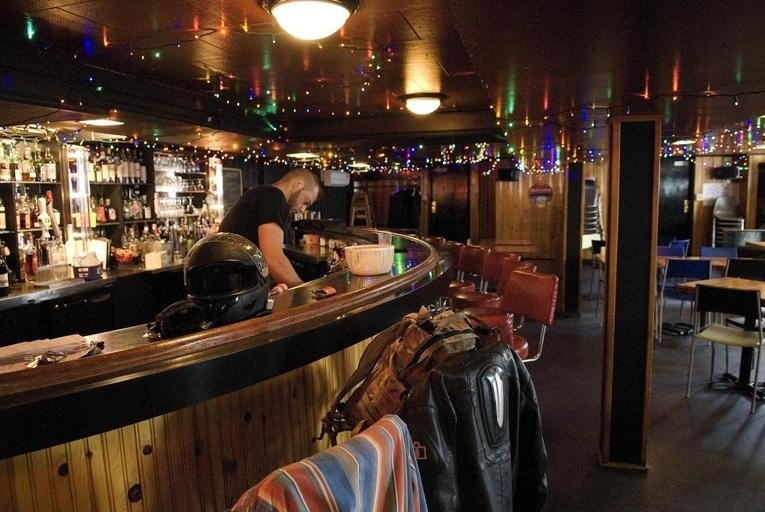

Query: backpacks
left=321, top=305, right=496, bottom=438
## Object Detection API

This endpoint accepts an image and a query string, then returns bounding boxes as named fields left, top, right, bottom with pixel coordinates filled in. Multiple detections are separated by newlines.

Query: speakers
left=716, top=166, right=740, bottom=181
left=497, top=168, right=521, bottom=181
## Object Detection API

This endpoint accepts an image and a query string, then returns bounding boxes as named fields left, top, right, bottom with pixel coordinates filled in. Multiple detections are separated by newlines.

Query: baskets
left=344, top=245, right=395, bottom=276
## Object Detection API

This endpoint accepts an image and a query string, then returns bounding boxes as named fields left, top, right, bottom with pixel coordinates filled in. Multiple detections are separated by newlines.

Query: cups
left=293, top=210, right=321, bottom=222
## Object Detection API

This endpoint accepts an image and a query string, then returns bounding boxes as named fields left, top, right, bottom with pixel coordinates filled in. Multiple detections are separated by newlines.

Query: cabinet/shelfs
left=0, top=132, right=215, bottom=279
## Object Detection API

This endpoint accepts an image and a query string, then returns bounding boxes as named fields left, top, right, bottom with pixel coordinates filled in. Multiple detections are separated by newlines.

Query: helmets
left=183, top=232, right=270, bottom=326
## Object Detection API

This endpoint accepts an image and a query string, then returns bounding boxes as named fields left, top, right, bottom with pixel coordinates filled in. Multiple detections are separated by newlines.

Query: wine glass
left=154, top=157, right=204, bottom=216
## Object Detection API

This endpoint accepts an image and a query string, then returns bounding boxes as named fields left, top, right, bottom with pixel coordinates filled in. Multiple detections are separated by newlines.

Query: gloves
left=149, top=298, right=207, bottom=339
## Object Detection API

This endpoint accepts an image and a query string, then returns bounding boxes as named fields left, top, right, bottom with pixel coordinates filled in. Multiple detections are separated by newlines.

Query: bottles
left=0, top=250, right=10, bottom=296
left=108, top=217, right=219, bottom=267
left=84, top=145, right=148, bottom=184
left=122, top=189, right=151, bottom=220
left=18, top=229, right=64, bottom=279
left=0, top=194, right=6, bottom=231
left=90, top=192, right=117, bottom=222
left=184, top=153, right=200, bottom=171
left=0, top=143, right=56, bottom=182
left=16, top=186, right=60, bottom=230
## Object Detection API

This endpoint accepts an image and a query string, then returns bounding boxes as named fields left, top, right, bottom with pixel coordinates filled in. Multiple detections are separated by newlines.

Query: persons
left=218, top=168, right=328, bottom=288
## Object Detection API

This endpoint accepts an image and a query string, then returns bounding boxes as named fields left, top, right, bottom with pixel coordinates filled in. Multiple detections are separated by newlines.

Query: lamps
left=395, top=92, right=448, bottom=116
left=258, top=0, right=362, bottom=42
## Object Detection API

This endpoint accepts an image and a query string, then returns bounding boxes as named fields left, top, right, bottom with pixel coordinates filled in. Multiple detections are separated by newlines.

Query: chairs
left=232, top=410, right=427, bottom=512
left=399, top=229, right=564, bottom=510
left=583, top=177, right=765, bottom=414
